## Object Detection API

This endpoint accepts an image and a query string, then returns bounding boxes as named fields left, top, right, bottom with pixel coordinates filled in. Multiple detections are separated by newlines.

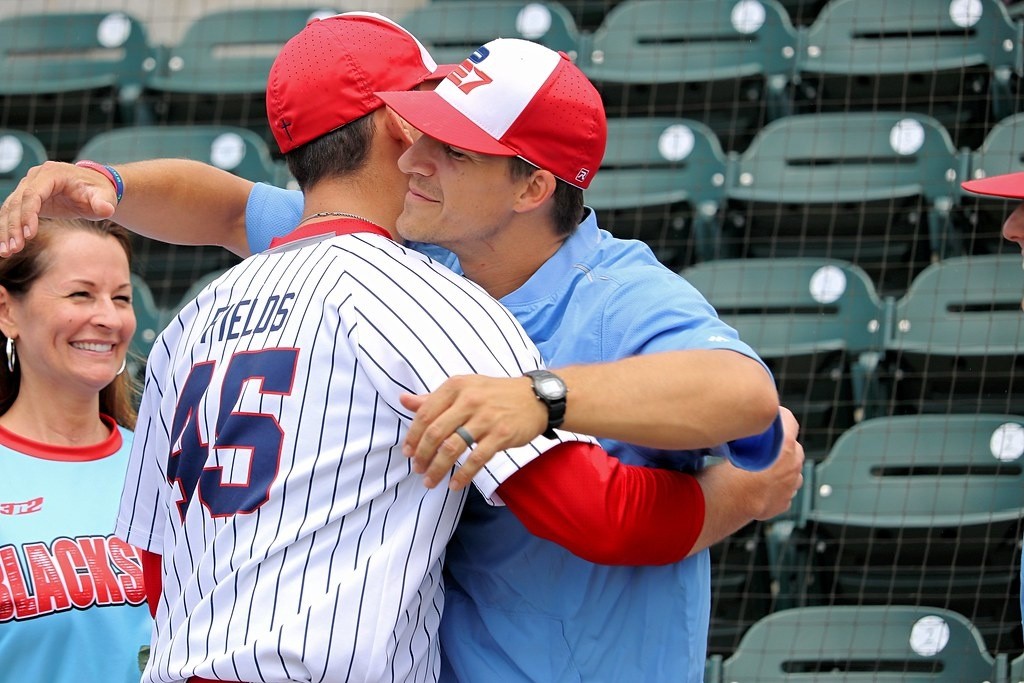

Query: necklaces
left=296, top=211, right=380, bottom=228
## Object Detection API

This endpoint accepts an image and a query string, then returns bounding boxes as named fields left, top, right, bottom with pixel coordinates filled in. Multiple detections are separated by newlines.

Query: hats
left=961, top=171, right=1024, bottom=201
left=374, top=38, right=608, bottom=189
left=266, top=11, right=460, bottom=155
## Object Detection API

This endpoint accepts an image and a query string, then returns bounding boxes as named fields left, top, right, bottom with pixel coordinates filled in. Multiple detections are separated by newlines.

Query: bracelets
left=76, top=160, right=124, bottom=205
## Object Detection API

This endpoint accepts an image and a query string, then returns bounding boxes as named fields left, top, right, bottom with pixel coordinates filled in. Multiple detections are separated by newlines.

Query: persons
left=961, top=172, right=1024, bottom=313
left=0, top=38, right=784, bottom=683
left=0, top=219, right=153, bottom=683
left=113, top=11, right=806, bottom=683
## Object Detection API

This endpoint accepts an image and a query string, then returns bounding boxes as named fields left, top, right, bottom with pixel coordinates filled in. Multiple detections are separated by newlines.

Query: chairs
left=583, top=116, right=729, bottom=264
left=964, top=114, right=1024, bottom=253
left=73, top=127, right=282, bottom=289
left=680, top=259, right=886, bottom=461
left=0, top=10, right=146, bottom=145
left=145, top=6, right=346, bottom=124
left=583, top=0, right=798, bottom=145
left=781, top=0, right=1024, bottom=123
left=705, top=605, right=1009, bottom=683
left=794, top=415, right=1024, bottom=635
left=398, top=0, right=584, bottom=69
left=716, top=112, right=971, bottom=297
left=873, top=253, right=1024, bottom=412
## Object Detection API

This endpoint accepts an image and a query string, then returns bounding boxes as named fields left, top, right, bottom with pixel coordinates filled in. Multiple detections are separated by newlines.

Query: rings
left=457, top=427, right=474, bottom=446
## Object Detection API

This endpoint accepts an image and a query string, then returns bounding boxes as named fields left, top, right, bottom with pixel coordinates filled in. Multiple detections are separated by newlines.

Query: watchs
left=523, top=370, right=567, bottom=439
